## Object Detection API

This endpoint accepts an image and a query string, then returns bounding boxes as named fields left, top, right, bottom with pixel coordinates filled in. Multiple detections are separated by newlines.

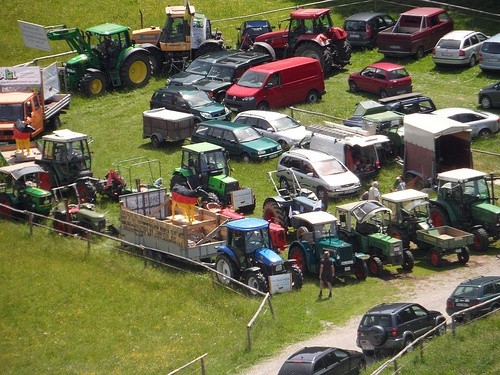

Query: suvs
left=356, top=302, right=447, bottom=357
left=195, top=51, right=274, bottom=105
left=445, top=275, right=500, bottom=322
left=165, top=48, right=243, bottom=89
left=149, top=85, right=233, bottom=124
left=276, top=148, right=362, bottom=199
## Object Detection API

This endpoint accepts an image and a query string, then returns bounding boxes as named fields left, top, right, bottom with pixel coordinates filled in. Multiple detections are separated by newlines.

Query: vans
left=227, top=56, right=326, bottom=113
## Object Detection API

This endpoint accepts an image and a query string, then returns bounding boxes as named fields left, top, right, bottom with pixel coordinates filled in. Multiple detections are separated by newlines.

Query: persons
left=368, top=181, right=381, bottom=222
left=318, top=251, right=335, bottom=298
left=394, top=175, right=405, bottom=218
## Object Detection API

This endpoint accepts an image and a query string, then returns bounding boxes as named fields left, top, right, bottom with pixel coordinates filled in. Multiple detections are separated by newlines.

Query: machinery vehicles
left=0, top=106, right=500, bottom=296
left=256, top=7, right=352, bottom=76
left=49, top=22, right=158, bottom=98
left=133, top=1, right=231, bottom=74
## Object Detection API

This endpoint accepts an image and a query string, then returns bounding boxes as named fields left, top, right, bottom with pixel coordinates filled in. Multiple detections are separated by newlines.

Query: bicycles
left=161, top=53, right=193, bottom=77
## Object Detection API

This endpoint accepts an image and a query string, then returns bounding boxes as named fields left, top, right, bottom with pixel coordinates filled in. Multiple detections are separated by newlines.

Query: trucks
left=0, top=91, right=71, bottom=142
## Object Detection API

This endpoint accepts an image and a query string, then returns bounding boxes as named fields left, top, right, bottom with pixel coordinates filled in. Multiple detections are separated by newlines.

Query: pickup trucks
left=376, top=8, right=454, bottom=62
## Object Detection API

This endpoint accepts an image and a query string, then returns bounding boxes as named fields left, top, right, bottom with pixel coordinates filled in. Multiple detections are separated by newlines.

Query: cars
left=478, top=82, right=500, bottom=109
left=192, top=121, right=282, bottom=161
left=235, top=111, right=312, bottom=150
left=400, top=105, right=500, bottom=140
left=434, top=29, right=489, bottom=69
left=235, top=19, right=276, bottom=49
left=343, top=11, right=396, bottom=48
left=479, top=33, right=500, bottom=71
left=278, top=347, right=366, bottom=375
left=347, top=62, right=413, bottom=98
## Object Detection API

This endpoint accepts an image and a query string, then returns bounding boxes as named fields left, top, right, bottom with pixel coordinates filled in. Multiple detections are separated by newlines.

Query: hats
left=396, top=175, right=403, bottom=179
left=323, top=252, right=329, bottom=258
